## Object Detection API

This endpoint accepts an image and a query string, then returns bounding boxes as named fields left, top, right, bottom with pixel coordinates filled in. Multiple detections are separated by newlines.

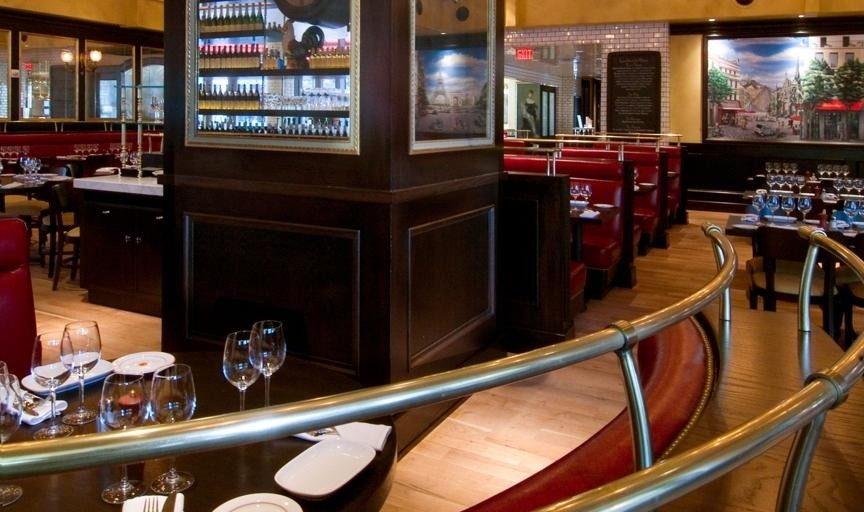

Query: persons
left=521, top=90, right=540, bottom=137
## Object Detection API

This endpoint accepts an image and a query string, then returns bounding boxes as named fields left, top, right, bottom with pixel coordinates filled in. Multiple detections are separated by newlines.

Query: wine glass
left=223, top=331, right=263, bottom=412
left=130, top=152, right=137, bottom=166
left=570, top=184, right=592, bottom=201
left=151, top=96, right=164, bottom=123
left=30, top=332, right=73, bottom=443
left=262, top=89, right=350, bottom=111
left=150, top=364, right=196, bottom=497
left=20, top=156, right=41, bottom=178
left=752, top=162, right=811, bottom=220
left=60, top=322, right=102, bottom=426
left=634, top=168, right=639, bottom=185
left=0, top=145, right=30, bottom=158
left=249, top=323, right=284, bottom=407
left=817, top=164, right=864, bottom=229
left=74, top=143, right=99, bottom=154
left=98, top=371, right=149, bottom=503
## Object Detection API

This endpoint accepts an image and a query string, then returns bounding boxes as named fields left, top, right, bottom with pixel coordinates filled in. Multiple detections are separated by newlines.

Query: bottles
left=265, top=47, right=283, bottom=70
left=267, top=21, right=281, bottom=31
left=198, top=117, right=350, bottom=137
left=198, top=2, right=264, bottom=116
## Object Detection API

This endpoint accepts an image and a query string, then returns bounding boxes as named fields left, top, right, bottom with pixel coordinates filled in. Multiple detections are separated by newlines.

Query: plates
left=21, top=358, right=113, bottom=397
left=570, top=200, right=614, bottom=209
left=211, top=437, right=377, bottom=512
left=639, top=183, right=655, bottom=186
left=763, top=215, right=797, bottom=221
left=309, top=46, right=349, bottom=69
left=111, top=350, right=176, bottom=377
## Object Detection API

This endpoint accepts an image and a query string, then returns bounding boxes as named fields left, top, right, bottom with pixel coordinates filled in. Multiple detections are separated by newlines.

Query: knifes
left=161, top=491, right=178, bottom=512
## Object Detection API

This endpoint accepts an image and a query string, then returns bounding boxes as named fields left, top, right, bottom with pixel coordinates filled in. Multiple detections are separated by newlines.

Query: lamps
left=85, top=49, right=103, bottom=72
left=59, top=48, right=76, bottom=72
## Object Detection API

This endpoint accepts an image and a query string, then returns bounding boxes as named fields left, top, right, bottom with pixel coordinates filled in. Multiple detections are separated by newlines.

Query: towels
left=1, top=383, right=69, bottom=426
left=292, top=422, right=393, bottom=452
left=121, top=494, right=185, bottom=511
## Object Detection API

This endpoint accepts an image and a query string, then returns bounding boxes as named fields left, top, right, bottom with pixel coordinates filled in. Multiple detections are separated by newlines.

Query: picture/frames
left=182, top=0, right=362, bottom=158
left=401, top=0, right=500, bottom=157
left=699, top=28, right=864, bottom=145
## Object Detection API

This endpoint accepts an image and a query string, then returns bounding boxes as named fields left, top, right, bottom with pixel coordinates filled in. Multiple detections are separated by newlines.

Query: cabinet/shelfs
left=517, top=84, right=557, bottom=155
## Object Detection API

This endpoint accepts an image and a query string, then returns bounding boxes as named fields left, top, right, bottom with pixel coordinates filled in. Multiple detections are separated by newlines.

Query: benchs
left=1, top=215, right=44, bottom=379
left=461, top=315, right=716, bottom=511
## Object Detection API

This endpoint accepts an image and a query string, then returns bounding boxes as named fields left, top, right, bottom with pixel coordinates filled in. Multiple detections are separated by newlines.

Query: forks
left=142, top=498, right=160, bottom=512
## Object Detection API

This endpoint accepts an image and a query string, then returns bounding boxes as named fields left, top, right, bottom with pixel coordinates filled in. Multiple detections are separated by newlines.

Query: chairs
left=0, top=150, right=165, bottom=292
left=750, top=224, right=864, bottom=350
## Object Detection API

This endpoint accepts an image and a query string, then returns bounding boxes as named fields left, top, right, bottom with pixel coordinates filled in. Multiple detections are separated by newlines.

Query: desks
left=69, top=173, right=162, bottom=320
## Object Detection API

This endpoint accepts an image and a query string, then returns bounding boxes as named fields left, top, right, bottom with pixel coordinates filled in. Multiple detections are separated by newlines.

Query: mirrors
left=0, top=6, right=165, bottom=134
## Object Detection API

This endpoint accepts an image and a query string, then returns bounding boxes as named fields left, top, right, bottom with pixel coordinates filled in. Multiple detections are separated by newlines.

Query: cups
left=1, top=360, right=22, bottom=444
